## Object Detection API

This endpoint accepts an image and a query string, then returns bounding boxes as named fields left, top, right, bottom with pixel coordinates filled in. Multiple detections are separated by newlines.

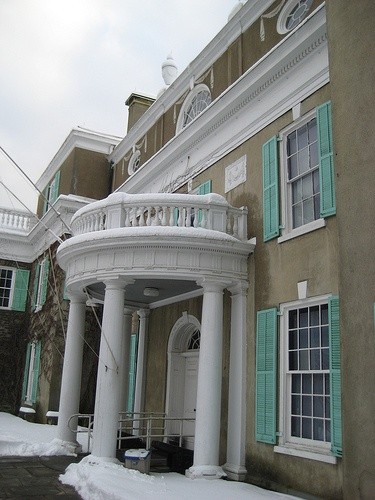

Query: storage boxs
left=124, top=449, right=152, bottom=477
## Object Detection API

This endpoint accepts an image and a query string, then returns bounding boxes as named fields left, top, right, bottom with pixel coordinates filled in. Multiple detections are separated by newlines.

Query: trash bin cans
left=124, top=449, right=151, bottom=475
